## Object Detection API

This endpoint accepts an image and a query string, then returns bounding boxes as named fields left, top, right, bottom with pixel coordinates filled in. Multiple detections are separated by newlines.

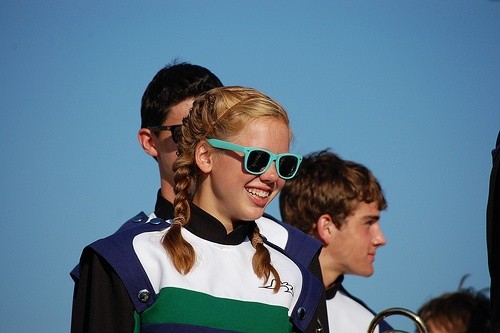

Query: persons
left=67, top=61, right=222, bottom=333
left=70, top=85, right=331, bottom=333
left=279, top=148, right=396, bottom=333
left=416, top=285, right=494, bottom=333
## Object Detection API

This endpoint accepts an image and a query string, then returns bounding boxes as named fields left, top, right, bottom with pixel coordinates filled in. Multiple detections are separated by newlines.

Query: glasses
left=152, top=124, right=185, bottom=144
left=206, top=137, right=303, bottom=180
